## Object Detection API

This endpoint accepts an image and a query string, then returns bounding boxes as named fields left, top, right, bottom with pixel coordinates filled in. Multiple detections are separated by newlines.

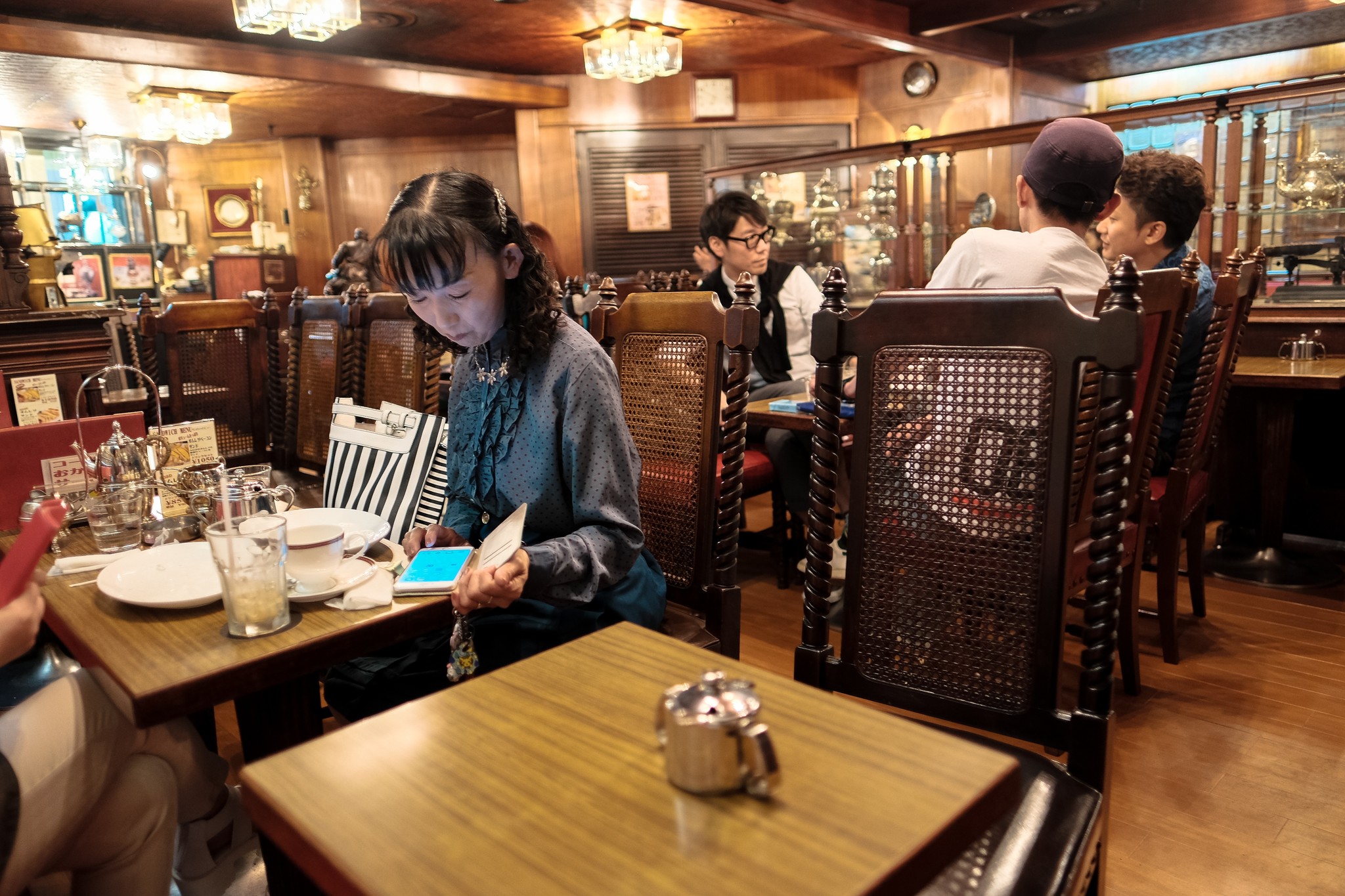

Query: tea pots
left=68, top=421, right=172, bottom=528
left=1275, top=139, right=1345, bottom=211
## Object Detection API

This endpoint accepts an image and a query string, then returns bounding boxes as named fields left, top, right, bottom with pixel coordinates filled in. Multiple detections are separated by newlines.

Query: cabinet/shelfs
left=213, top=253, right=299, bottom=300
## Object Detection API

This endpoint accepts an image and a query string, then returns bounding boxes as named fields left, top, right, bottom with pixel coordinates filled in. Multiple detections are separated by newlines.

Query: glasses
left=726, top=225, right=776, bottom=249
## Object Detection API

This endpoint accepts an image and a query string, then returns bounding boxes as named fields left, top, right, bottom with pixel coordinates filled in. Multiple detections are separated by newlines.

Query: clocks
left=690, top=73, right=738, bottom=121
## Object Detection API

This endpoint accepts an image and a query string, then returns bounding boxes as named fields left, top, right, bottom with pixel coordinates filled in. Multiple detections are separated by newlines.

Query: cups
left=654, top=671, right=783, bottom=799
left=177, top=456, right=227, bottom=513
left=1278, top=333, right=1327, bottom=362
left=802, top=366, right=857, bottom=400
left=203, top=515, right=291, bottom=638
left=219, top=466, right=271, bottom=489
left=284, top=524, right=368, bottom=593
left=81, top=492, right=143, bottom=554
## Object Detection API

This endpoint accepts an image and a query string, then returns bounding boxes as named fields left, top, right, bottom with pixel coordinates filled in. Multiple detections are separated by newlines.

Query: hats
left=1022, top=116, right=1123, bottom=207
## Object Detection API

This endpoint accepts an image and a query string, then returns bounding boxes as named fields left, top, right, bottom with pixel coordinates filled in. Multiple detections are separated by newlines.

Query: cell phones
left=393, top=547, right=477, bottom=593
left=796, top=402, right=854, bottom=419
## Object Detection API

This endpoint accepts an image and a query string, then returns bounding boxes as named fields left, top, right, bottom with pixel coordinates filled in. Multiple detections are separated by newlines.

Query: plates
left=238, top=508, right=391, bottom=555
left=286, top=553, right=378, bottom=603
left=96, top=539, right=251, bottom=610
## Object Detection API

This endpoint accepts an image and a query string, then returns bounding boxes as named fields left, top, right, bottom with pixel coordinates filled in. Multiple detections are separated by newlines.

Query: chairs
left=148, top=245, right=1266, bottom=896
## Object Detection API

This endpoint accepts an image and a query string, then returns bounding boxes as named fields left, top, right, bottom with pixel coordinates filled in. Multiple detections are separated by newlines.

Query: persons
left=654, top=117, right=1216, bottom=579
left=0, top=576, right=264, bottom=896
left=371, top=174, right=669, bottom=687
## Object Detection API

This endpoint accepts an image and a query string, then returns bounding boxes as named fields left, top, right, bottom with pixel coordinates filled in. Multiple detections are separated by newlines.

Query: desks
left=0, top=499, right=450, bottom=766
left=746, top=392, right=904, bottom=434
left=238, top=622, right=1021, bottom=896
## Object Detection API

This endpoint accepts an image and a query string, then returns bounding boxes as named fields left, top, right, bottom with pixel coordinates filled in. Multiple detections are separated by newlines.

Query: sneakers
left=797, top=539, right=847, bottom=579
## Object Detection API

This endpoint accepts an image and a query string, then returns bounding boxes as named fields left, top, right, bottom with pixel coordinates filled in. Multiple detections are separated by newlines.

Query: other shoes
left=171, top=784, right=261, bottom=896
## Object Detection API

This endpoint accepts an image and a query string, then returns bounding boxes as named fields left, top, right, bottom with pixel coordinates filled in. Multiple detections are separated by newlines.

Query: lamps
left=0, top=126, right=123, bottom=195
left=573, top=17, right=691, bottom=84
left=232, top=0, right=362, bottom=43
left=131, top=85, right=236, bottom=145
